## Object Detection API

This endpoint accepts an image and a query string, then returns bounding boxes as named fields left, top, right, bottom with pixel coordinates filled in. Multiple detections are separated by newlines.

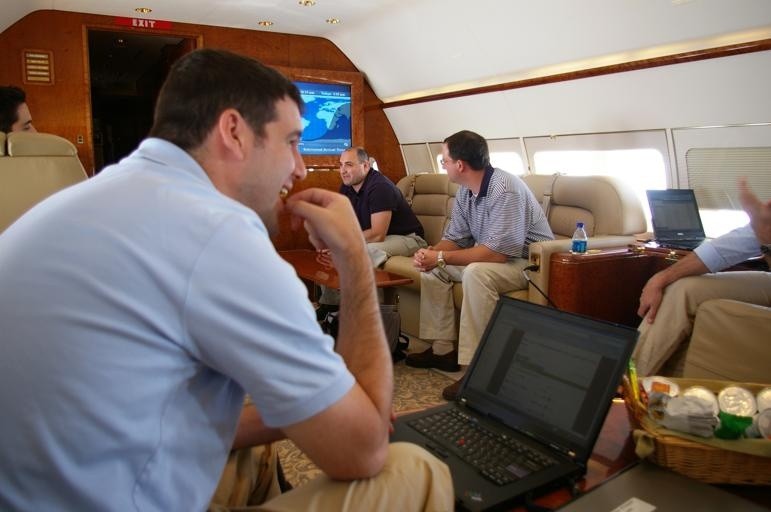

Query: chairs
left=1, top=131, right=90, bottom=230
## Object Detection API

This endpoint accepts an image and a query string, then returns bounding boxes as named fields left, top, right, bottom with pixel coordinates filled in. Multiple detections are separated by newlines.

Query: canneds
left=641, top=375, right=771, bottom=440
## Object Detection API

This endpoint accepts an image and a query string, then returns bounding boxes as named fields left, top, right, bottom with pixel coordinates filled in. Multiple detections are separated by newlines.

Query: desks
left=278, top=248, right=414, bottom=363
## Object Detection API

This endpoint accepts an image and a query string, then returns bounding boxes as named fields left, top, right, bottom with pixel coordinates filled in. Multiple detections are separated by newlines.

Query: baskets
left=622, top=374, right=771, bottom=486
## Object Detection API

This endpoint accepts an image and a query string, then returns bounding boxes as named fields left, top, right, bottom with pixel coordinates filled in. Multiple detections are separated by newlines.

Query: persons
left=314, top=146, right=423, bottom=333
left=408, top=128, right=558, bottom=399
left=0, top=46, right=456, bottom=511
left=608, top=171, right=771, bottom=377
left=1, top=84, right=40, bottom=133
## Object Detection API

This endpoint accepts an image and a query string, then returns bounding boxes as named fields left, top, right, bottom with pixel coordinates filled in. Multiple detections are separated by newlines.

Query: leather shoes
left=406, top=346, right=460, bottom=371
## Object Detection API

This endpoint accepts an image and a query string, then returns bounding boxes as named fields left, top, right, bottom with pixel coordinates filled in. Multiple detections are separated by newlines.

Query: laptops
left=646, top=188, right=710, bottom=250
left=390, top=294, right=639, bottom=512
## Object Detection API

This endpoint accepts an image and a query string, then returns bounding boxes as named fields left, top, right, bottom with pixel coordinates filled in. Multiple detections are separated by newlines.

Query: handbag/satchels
left=325, top=314, right=409, bottom=362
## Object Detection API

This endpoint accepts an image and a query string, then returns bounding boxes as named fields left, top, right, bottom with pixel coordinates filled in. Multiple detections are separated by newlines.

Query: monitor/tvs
left=289, top=79, right=353, bottom=155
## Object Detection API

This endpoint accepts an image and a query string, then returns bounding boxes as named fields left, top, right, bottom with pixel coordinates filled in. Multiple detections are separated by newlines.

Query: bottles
left=569, top=222, right=588, bottom=257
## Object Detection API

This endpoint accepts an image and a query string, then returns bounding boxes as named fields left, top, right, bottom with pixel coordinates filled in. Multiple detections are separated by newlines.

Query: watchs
left=760, top=242, right=771, bottom=256
left=438, top=249, right=447, bottom=268
